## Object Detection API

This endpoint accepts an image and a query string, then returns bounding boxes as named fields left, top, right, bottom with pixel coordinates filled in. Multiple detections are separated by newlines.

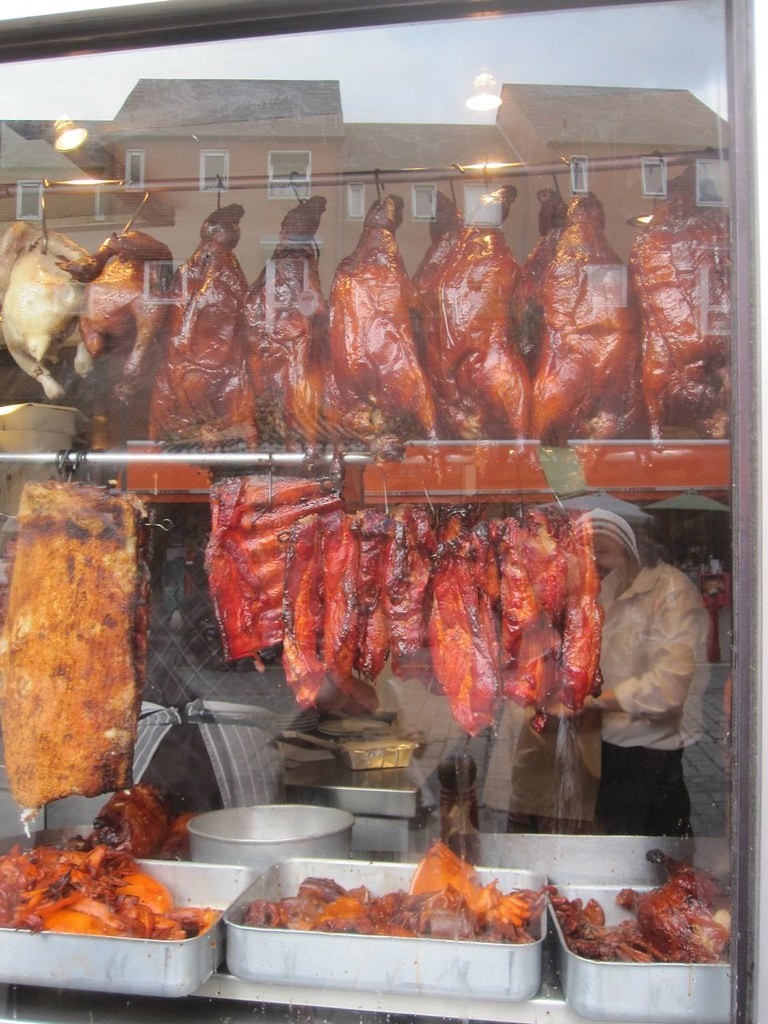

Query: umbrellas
left=543, top=489, right=734, bottom=525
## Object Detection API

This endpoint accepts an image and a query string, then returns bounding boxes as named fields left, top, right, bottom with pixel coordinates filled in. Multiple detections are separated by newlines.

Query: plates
left=269, top=708, right=317, bottom=735
left=318, top=719, right=390, bottom=737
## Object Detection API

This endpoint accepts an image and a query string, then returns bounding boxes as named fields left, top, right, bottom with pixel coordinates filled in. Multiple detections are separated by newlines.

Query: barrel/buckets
left=186, top=805, right=355, bottom=867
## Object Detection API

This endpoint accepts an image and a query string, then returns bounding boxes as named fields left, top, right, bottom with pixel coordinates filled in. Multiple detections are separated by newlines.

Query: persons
left=484, top=696, right=602, bottom=834
left=545, top=509, right=715, bottom=835
left=131, top=490, right=377, bottom=824
